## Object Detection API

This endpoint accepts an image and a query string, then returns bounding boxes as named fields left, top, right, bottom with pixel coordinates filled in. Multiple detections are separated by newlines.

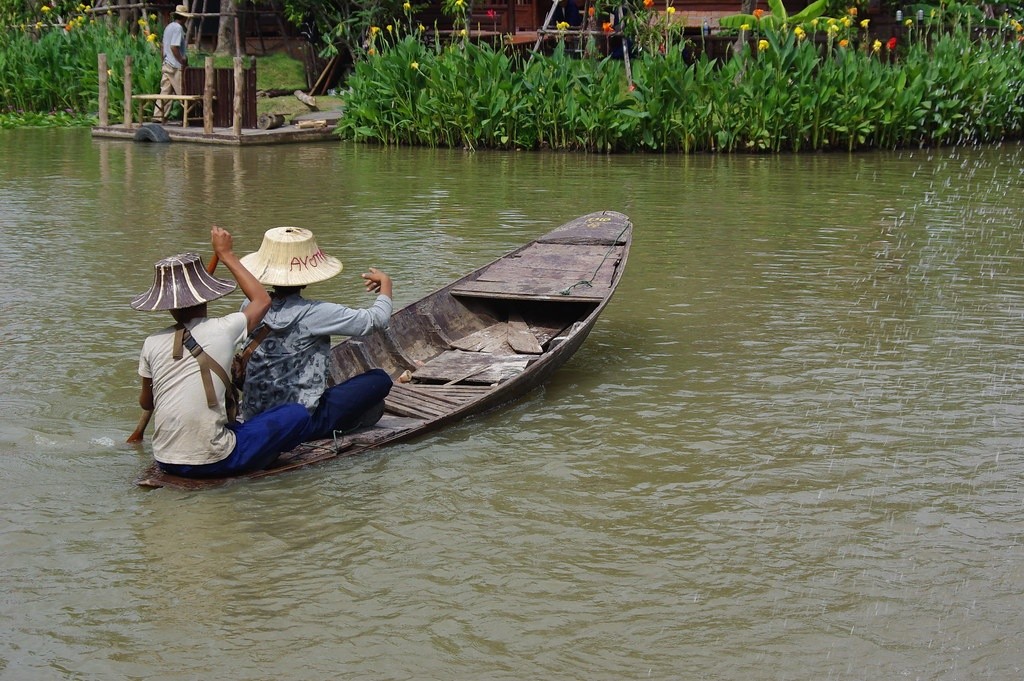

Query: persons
left=131, top=225, right=313, bottom=479
left=239, top=226, right=393, bottom=442
left=151, top=4, right=194, bottom=124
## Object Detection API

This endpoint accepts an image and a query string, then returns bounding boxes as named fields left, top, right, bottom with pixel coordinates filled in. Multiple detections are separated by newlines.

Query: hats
left=130, top=253, right=237, bottom=311
left=239, top=226, right=343, bottom=286
left=170, top=4, right=194, bottom=17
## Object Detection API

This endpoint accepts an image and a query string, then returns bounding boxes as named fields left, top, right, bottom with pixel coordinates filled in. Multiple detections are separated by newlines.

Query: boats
left=137, top=209, right=633, bottom=490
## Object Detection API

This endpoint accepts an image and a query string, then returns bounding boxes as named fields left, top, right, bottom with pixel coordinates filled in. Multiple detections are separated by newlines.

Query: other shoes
left=329, top=399, right=384, bottom=435
left=151, top=116, right=163, bottom=122
